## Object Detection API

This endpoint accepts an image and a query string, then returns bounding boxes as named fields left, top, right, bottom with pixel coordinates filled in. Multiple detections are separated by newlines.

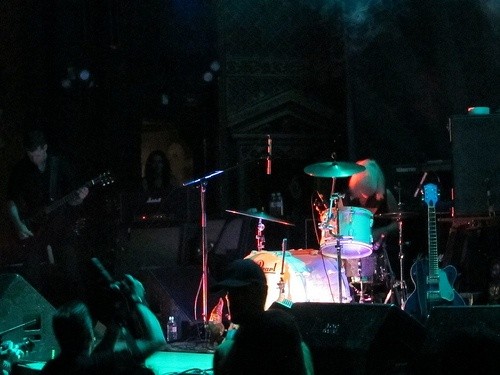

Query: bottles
left=486, top=265, right=500, bottom=306
left=271, top=192, right=283, bottom=217
left=167, top=316, right=178, bottom=341
left=484, top=178, right=495, bottom=217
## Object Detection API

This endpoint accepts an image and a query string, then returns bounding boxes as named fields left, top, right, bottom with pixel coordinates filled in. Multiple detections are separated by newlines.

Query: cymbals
left=305, top=162, right=366, bottom=178
left=226, top=207, right=295, bottom=229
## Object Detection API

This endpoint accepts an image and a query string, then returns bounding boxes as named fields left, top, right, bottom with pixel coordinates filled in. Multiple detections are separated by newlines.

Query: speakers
left=421, top=304, right=500, bottom=375
left=288, top=302, right=425, bottom=375
left=0, top=272, right=63, bottom=363
left=449, top=113, right=500, bottom=217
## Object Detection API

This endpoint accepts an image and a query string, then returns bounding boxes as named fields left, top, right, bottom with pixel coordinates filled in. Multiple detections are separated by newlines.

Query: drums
left=239, top=248, right=353, bottom=311
left=346, top=255, right=375, bottom=284
left=318, top=206, right=373, bottom=259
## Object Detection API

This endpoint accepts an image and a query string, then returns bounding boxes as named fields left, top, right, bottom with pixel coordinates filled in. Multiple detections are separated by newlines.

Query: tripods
left=381, top=216, right=414, bottom=309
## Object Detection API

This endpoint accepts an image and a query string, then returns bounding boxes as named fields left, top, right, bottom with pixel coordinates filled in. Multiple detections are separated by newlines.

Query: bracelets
left=131, top=297, right=144, bottom=303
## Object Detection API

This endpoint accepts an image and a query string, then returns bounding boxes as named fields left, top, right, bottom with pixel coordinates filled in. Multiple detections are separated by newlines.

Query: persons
left=38, top=273, right=165, bottom=375
left=8, top=129, right=88, bottom=274
left=268, top=172, right=319, bottom=249
left=341, top=158, right=402, bottom=301
left=209, top=259, right=313, bottom=375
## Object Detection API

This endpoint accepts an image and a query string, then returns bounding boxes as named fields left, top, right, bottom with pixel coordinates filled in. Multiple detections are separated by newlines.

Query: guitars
left=0, top=171, right=115, bottom=256
left=404, top=182, right=469, bottom=333
